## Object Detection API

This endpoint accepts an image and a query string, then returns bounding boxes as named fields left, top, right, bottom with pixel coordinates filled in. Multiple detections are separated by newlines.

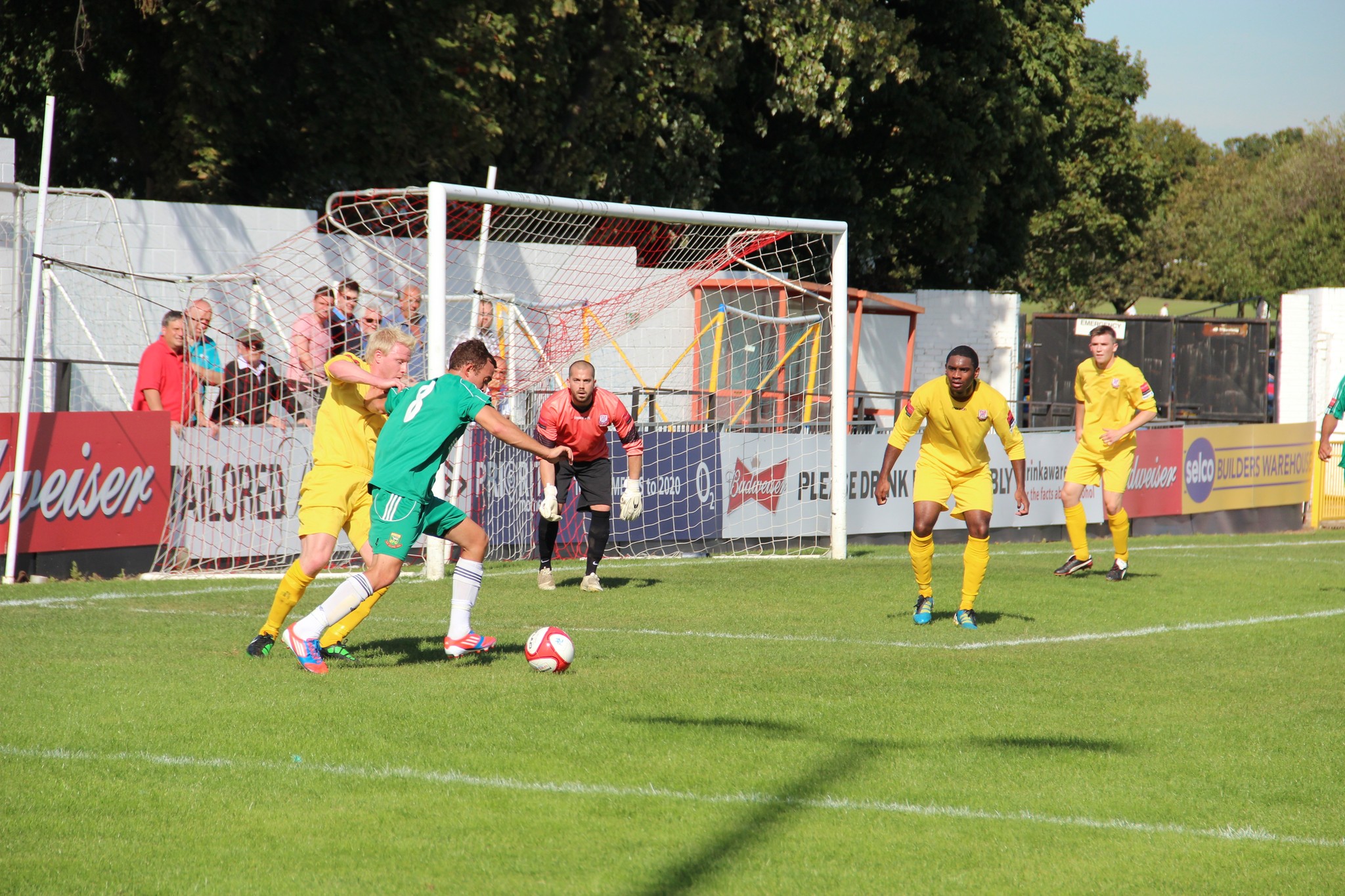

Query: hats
left=237, top=328, right=264, bottom=342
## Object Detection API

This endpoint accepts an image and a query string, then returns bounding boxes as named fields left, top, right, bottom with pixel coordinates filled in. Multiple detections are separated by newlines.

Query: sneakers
left=912, top=594, right=934, bottom=625
left=952, top=608, right=977, bottom=630
left=281, top=621, right=329, bottom=674
left=245, top=632, right=274, bottom=659
left=537, top=567, right=556, bottom=590
left=1105, top=557, right=1129, bottom=581
left=444, top=629, right=497, bottom=658
left=318, top=639, right=359, bottom=662
left=1053, top=553, right=1093, bottom=577
left=580, top=571, right=604, bottom=592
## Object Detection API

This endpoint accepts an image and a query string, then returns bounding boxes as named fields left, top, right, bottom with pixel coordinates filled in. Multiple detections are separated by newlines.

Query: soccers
left=524, top=626, right=574, bottom=673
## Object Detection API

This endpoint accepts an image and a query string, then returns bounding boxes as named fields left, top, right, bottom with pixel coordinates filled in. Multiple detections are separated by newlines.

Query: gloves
left=539, top=483, right=563, bottom=522
left=619, top=476, right=642, bottom=521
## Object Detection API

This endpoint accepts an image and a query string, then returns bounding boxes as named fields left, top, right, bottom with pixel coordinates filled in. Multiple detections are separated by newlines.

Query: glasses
left=365, top=318, right=381, bottom=324
left=342, top=295, right=357, bottom=301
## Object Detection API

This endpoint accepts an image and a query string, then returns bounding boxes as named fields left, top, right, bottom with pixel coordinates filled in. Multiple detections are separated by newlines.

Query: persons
left=1126, top=301, right=1137, bottom=316
left=131, top=311, right=218, bottom=439
left=536, top=360, right=644, bottom=592
left=445, top=299, right=500, bottom=375
left=282, top=340, right=573, bottom=676
left=358, top=303, right=382, bottom=353
left=184, top=300, right=225, bottom=426
left=1053, top=326, right=1157, bottom=581
left=279, top=286, right=335, bottom=427
left=1160, top=302, right=1168, bottom=316
left=246, top=326, right=415, bottom=661
left=213, top=328, right=315, bottom=430
left=1318, top=375, right=1345, bottom=480
left=876, top=345, right=1030, bottom=630
left=486, top=355, right=508, bottom=410
left=322, top=279, right=363, bottom=358
left=381, top=285, right=427, bottom=384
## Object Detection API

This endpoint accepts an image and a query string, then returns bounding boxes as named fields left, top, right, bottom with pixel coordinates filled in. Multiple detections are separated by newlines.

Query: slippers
left=245, top=343, right=262, bottom=350
